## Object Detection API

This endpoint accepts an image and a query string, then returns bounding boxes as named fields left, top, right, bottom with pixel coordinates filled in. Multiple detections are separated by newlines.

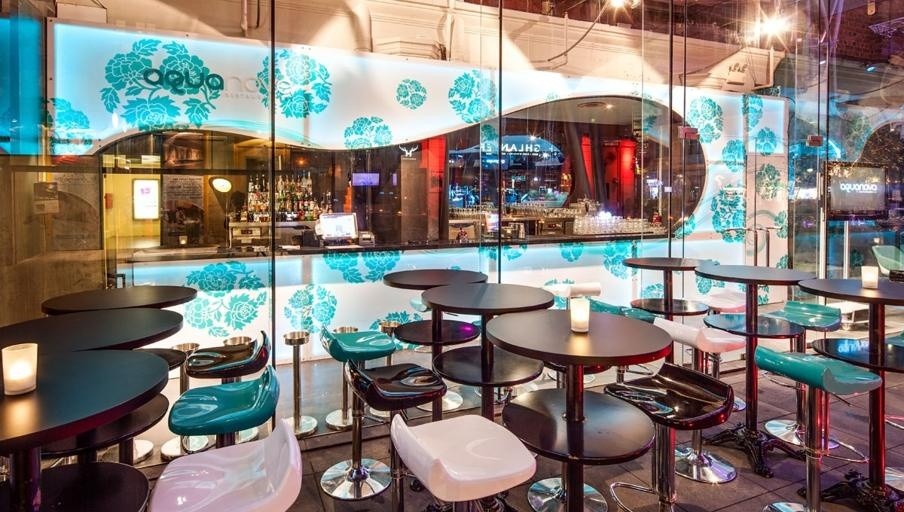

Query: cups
left=569, top=333, right=591, bottom=358
left=0, top=344, right=41, bottom=395
left=569, top=296, right=591, bottom=334
left=569, top=214, right=649, bottom=234
left=861, top=288, right=878, bottom=298
left=3, top=396, right=38, bottom=438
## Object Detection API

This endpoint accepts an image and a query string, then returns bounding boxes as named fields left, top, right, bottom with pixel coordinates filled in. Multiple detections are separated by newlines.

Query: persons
left=520, top=177, right=543, bottom=202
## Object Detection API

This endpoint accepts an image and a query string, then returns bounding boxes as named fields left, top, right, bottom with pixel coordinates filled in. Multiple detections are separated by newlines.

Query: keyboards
left=324, top=245, right=363, bottom=249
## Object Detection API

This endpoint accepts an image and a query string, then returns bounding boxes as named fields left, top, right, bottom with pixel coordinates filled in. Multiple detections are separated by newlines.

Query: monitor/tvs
left=320, top=212, right=359, bottom=241
left=352, top=172, right=381, bottom=186
left=824, top=160, right=888, bottom=220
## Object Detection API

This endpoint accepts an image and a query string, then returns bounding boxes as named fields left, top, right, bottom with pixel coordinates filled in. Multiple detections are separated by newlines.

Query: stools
left=604, top=362, right=739, bottom=511
left=337, top=356, right=451, bottom=512
left=656, top=325, right=748, bottom=487
left=166, top=364, right=281, bottom=446
left=702, top=289, right=748, bottom=413
left=586, top=297, right=659, bottom=380
left=871, top=244, right=904, bottom=349
left=384, top=406, right=545, bottom=511
left=181, top=327, right=279, bottom=450
left=751, top=344, right=883, bottom=512
left=314, top=325, right=402, bottom=503
left=143, top=418, right=305, bottom=511
left=757, top=299, right=844, bottom=452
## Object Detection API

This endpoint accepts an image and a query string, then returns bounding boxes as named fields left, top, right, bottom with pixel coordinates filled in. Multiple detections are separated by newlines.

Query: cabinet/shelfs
left=225, top=174, right=336, bottom=248
left=446, top=192, right=669, bottom=242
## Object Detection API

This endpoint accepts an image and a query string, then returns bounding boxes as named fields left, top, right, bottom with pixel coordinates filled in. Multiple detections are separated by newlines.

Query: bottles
left=248, top=169, right=333, bottom=222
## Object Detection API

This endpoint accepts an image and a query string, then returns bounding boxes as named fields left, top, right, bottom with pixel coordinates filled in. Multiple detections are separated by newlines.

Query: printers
left=358, top=231, right=375, bottom=245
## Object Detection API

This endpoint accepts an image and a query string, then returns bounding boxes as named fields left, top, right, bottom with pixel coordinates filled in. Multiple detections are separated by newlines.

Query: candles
left=7, top=363, right=34, bottom=392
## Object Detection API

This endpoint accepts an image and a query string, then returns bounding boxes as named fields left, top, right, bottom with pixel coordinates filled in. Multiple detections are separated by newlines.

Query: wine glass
left=541, top=206, right=583, bottom=218
left=451, top=201, right=548, bottom=219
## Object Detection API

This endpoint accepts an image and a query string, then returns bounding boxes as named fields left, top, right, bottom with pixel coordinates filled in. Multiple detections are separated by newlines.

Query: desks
left=798, top=277, right=904, bottom=512
left=692, top=262, right=819, bottom=479
left=1, top=347, right=170, bottom=510
left=621, top=255, right=721, bottom=367
left=42, top=284, right=199, bottom=467
left=0, top=308, right=185, bottom=467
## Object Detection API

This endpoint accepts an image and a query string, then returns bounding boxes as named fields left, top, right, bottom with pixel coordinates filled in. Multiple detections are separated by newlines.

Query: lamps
left=204, top=176, right=237, bottom=201
